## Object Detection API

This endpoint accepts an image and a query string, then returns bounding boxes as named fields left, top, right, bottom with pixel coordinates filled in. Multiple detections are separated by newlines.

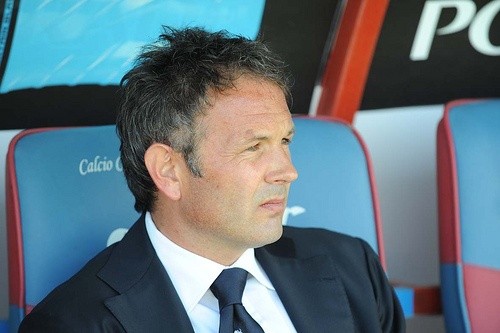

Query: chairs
left=6, top=114, right=389, bottom=333
left=438, top=99, right=500, bottom=333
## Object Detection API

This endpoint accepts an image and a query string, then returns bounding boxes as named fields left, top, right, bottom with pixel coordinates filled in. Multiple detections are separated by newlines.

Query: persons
left=15, top=22, right=409, bottom=333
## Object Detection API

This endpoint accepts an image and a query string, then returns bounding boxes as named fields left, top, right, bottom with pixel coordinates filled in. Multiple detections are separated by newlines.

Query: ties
left=207, top=267, right=265, bottom=333
